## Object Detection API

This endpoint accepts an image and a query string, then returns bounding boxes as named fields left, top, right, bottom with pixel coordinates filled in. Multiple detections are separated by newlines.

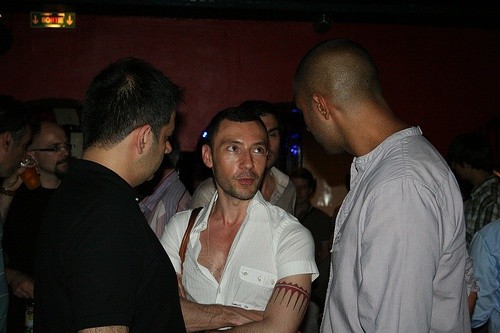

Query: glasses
left=33, top=142, right=75, bottom=154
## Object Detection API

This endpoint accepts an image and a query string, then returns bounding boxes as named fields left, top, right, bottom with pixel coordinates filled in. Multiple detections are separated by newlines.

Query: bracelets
left=0, top=186, right=15, bottom=196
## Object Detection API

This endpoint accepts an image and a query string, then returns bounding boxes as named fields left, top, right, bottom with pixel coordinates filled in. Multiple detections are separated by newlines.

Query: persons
left=293, top=36, right=471, bottom=333
left=448, top=131, right=500, bottom=333
left=0, top=56, right=332, bottom=333
